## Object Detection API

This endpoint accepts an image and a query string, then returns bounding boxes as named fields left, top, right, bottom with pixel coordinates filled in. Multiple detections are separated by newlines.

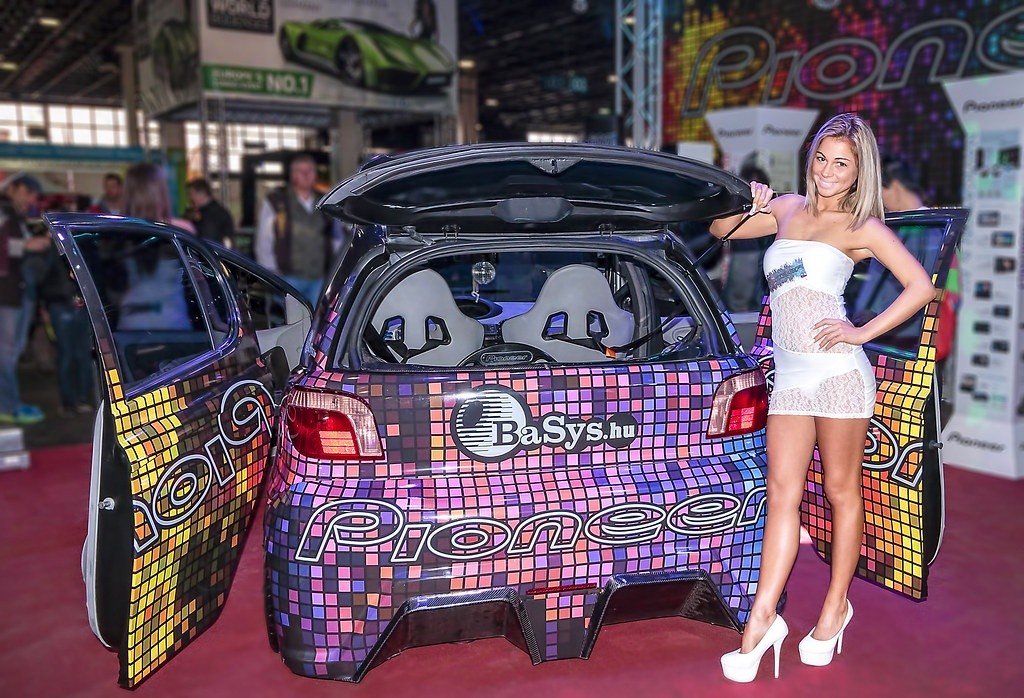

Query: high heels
left=799, top=599, right=855, bottom=667
left=721, top=609, right=790, bottom=682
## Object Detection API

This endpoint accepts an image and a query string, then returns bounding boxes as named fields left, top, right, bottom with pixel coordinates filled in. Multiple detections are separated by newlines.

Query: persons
left=709, top=113, right=960, bottom=683
left=253, top=153, right=344, bottom=311
left=0, top=172, right=234, bottom=423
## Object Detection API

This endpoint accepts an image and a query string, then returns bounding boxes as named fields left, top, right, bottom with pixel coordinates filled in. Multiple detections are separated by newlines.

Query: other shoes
left=63, top=399, right=92, bottom=415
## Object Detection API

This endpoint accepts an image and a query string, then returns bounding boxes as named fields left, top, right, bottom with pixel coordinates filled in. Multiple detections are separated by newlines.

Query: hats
left=14, top=173, right=47, bottom=202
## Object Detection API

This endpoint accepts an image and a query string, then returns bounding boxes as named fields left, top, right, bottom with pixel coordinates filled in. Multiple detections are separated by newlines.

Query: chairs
left=501, top=264, right=637, bottom=365
left=364, top=269, right=485, bottom=367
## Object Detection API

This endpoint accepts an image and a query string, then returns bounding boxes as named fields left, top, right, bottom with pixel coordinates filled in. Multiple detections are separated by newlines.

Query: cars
left=277, top=18, right=455, bottom=95
left=41, top=140, right=974, bottom=691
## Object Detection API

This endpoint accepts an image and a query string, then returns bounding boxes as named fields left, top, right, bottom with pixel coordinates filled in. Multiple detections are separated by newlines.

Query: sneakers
left=1, top=402, right=45, bottom=425
